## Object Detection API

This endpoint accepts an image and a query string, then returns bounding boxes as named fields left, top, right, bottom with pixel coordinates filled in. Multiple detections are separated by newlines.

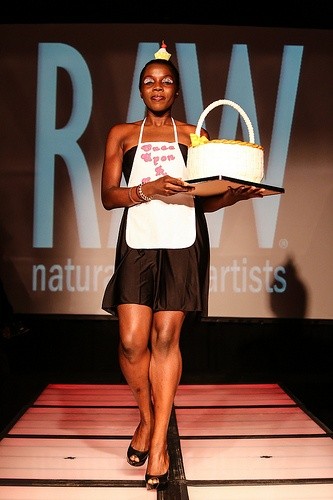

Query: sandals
left=126, top=442, right=149, bottom=467
left=144, top=445, right=170, bottom=492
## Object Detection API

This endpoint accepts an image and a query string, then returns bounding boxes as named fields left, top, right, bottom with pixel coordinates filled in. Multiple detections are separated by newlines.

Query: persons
left=101, top=40, right=265, bottom=489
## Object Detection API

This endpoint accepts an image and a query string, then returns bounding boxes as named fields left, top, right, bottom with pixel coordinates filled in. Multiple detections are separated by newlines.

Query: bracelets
left=128, top=185, right=152, bottom=204
